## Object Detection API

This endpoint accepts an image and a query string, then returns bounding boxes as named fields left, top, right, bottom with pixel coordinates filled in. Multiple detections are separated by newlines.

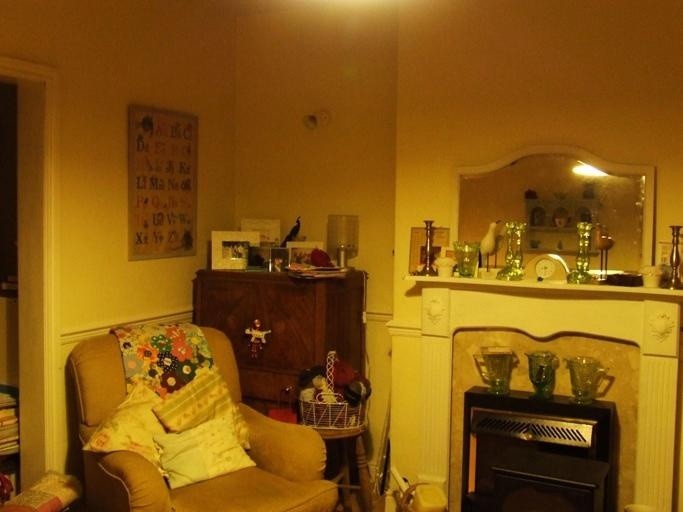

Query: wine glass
left=523, top=349, right=561, bottom=399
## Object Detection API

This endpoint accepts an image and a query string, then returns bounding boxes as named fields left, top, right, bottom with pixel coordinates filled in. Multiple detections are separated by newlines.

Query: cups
left=472, top=346, right=519, bottom=404
left=565, top=353, right=609, bottom=403
left=641, top=265, right=665, bottom=288
left=452, top=241, right=482, bottom=279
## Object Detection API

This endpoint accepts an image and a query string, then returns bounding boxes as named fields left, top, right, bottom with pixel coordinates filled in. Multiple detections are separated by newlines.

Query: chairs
left=65, top=321, right=340, bottom=511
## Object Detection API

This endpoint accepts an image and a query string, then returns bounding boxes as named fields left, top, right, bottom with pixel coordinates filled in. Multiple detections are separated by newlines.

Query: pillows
left=79, top=367, right=256, bottom=490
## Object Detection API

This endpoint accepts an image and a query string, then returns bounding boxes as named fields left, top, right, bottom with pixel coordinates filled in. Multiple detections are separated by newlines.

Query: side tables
left=315, top=418, right=373, bottom=511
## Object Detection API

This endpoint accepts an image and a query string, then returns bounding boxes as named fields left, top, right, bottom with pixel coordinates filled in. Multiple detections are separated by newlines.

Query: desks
left=191, top=265, right=369, bottom=403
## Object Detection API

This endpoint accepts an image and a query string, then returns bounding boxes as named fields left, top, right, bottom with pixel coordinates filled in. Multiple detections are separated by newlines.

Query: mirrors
left=448, top=143, right=656, bottom=279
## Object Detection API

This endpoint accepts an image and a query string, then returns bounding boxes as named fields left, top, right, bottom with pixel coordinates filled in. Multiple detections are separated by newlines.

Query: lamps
left=327, top=214, right=359, bottom=273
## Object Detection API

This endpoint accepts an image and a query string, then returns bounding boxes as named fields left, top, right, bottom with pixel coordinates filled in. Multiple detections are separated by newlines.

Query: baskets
left=296, top=392, right=370, bottom=431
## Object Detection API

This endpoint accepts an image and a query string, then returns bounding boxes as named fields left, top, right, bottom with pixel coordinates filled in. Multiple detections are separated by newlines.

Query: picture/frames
left=211, top=230, right=260, bottom=271
left=285, top=240, right=325, bottom=269
left=127, top=105, right=198, bottom=261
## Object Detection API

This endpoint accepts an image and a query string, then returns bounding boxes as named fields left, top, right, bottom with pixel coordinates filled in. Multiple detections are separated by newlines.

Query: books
left=287, top=263, right=347, bottom=281
left=0, top=392, right=19, bottom=456
